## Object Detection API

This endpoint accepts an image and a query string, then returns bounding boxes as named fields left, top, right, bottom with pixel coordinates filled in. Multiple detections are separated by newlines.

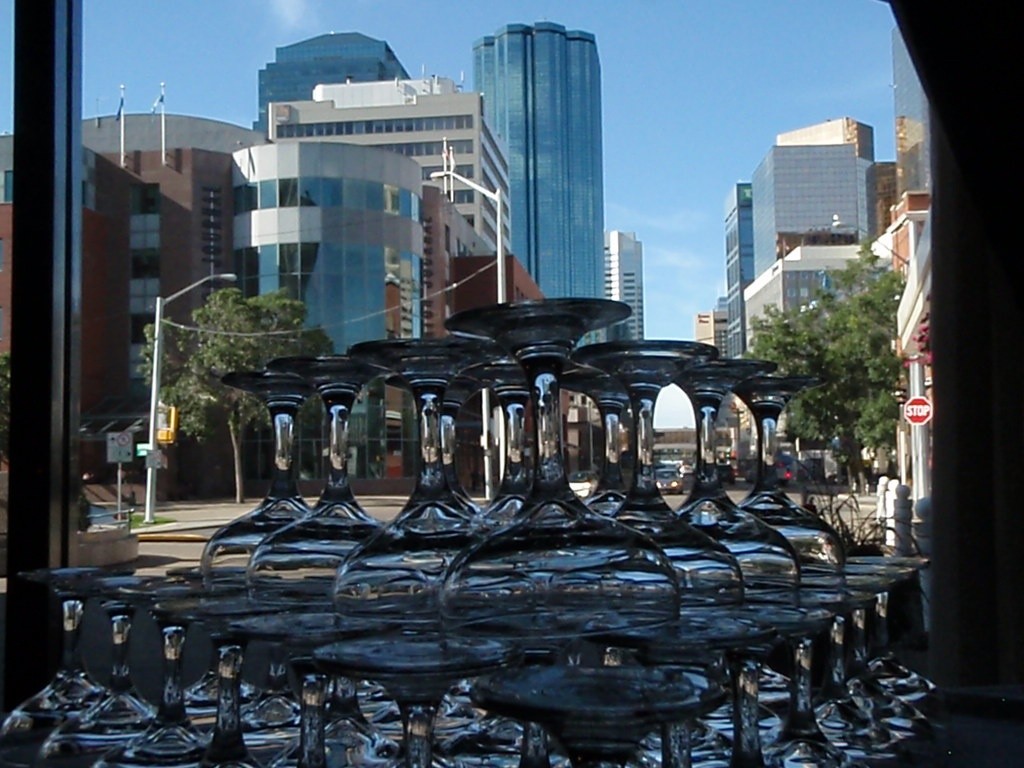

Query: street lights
left=831, top=218, right=909, bottom=266
left=428, top=170, right=510, bottom=484
left=142, top=270, right=241, bottom=523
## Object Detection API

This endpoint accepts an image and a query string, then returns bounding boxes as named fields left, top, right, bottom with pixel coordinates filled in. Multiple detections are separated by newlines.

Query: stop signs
left=904, top=397, right=934, bottom=423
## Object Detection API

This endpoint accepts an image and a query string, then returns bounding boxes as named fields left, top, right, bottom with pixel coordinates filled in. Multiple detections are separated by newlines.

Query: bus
left=659, top=461, right=684, bottom=468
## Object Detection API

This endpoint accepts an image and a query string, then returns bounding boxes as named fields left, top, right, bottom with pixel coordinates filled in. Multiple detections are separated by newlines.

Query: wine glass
left=0, top=290, right=955, bottom=768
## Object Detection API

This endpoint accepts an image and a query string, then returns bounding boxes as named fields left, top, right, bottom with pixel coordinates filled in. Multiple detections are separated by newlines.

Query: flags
left=115, top=91, right=124, bottom=121
left=151, top=94, right=164, bottom=113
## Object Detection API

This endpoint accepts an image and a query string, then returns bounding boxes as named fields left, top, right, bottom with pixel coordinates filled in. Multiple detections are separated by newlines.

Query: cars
left=656, top=469, right=684, bottom=494
left=678, top=465, right=695, bottom=474
left=748, top=462, right=791, bottom=484
left=581, top=489, right=627, bottom=518
left=719, top=462, right=736, bottom=485
left=568, top=471, right=600, bottom=496
